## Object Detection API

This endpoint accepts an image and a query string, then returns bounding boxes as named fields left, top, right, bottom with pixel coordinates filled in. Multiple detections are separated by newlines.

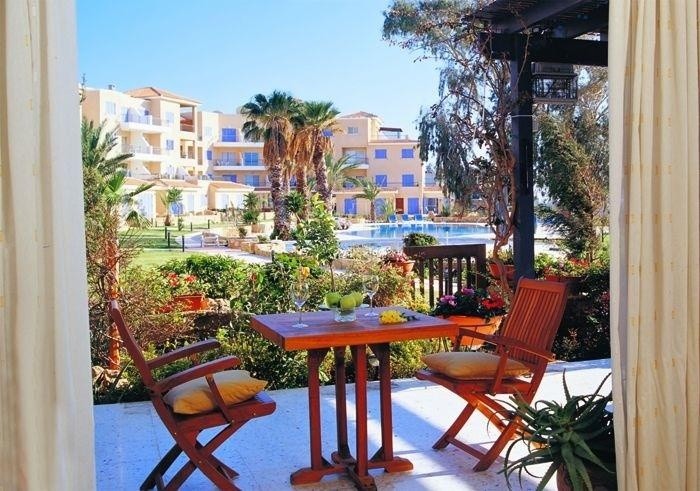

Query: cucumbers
left=351, top=291, right=363, bottom=307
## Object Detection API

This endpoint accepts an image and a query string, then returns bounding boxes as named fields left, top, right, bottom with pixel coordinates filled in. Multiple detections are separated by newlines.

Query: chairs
left=388, top=214, right=398, bottom=223
left=249, top=305, right=460, bottom=491
left=109, top=300, right=276, bottom=490
left=416, top=276, right=571, bottom=471
left=414, top=214, right=423, bottom=221
left=401, top=213, right=411, bottom=223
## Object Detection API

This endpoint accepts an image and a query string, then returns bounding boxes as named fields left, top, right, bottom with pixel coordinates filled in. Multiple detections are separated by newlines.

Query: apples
left=325, top=292, right=341, bottom=308
left=340, top=294, right=357, bottom=311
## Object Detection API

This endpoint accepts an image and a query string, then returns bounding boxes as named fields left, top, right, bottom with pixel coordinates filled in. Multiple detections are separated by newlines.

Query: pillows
left=161, top=368, right=268, bottom=414
left=418, top=350, right=531, bottom=381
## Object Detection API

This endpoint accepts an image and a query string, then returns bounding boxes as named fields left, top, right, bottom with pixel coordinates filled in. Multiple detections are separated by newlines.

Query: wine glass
left=288, top=282, right=310, bottom=330
left=362, top=274, right=381, bottom=317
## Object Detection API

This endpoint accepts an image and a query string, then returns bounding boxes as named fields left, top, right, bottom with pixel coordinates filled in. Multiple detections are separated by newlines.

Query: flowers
left=167, top=271, right=203, bottom=295
left=429, top=286, right=508, bottom=319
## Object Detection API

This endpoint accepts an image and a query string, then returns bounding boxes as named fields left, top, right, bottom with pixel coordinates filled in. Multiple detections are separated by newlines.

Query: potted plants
left=489, top=249, right=515, bottom=279
left=485, top=370, right=615, bottom=490
left=544, top=258, right=582, bottom=283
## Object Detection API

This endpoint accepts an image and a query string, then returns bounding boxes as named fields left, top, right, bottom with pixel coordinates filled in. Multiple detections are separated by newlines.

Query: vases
left=445, top=313, right=502, bottom=348
left=175, top=294, right=203, bottom=311
